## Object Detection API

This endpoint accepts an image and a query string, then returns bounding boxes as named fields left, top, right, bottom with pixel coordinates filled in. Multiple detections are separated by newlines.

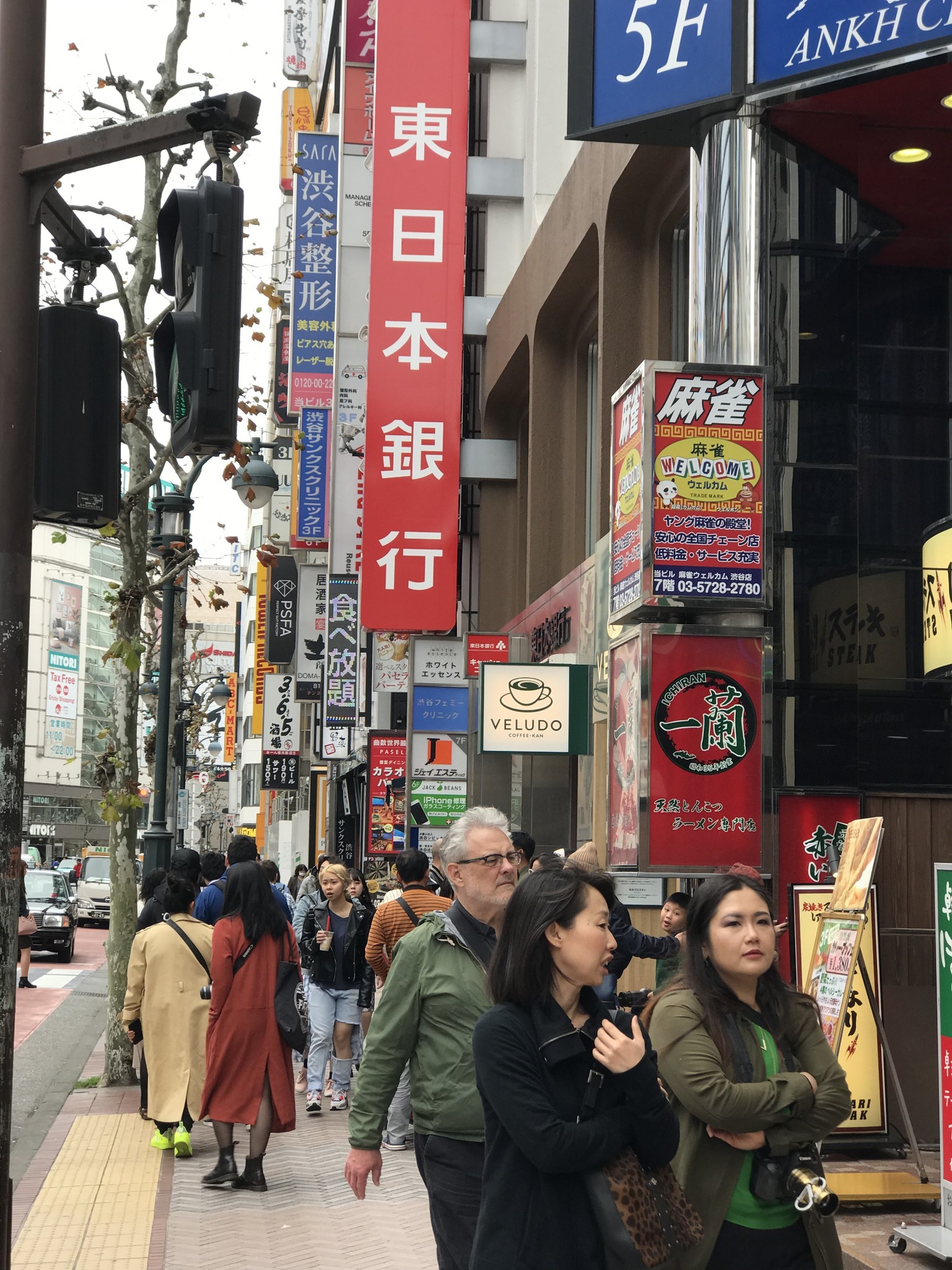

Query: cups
left=320, top=930, right=335, bottom=951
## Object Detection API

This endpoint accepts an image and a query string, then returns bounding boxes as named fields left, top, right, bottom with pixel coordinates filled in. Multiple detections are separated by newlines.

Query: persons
left=730, top=862, right=791, bottom=966
left=51, top=859, right=59, bottom=870
left=73, top=858, right=81, bottom=892
left=646, top=874, right=852, bottom=1270
left=470, top=857, right=681, bottom=1270
left=344, top=805, right=528, bottom=1269
left=508, top=832, right=691, bottom=1012
left=19, top=859, right=37, bottom=988
left=117, top=833, right=455, bottom=1194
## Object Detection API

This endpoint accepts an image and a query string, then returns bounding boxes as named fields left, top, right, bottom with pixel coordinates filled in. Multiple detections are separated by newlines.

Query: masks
left=299, top=872, right=306, bottom=877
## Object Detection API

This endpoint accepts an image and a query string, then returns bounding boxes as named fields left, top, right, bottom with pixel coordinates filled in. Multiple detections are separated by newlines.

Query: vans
left=27, top=846, right=42, bottom=869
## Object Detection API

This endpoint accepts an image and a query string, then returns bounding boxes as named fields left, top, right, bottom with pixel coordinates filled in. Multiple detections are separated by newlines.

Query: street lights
left=141, top=673, right=232, bottom=850
left=133, top=436, right=282, bottom=906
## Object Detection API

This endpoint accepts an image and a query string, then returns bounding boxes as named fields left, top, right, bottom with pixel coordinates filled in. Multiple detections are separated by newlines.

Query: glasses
left=457, top=852, right=521, bottom=868
left=528, top=869, right=534, bottom=874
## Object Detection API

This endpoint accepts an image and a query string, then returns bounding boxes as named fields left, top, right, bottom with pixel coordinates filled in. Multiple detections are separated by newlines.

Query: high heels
left=19, top=977, right=36, bottom=988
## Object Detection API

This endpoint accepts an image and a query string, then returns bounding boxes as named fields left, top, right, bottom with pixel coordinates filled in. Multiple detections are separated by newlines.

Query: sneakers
left=150, top=1128, right=174, bottom=1150
left=174, top=1121, right=193, bottom=1158
left=330, top=1088, right=348, bottom=1110
left=306, top=1088, right=321, bottom=1114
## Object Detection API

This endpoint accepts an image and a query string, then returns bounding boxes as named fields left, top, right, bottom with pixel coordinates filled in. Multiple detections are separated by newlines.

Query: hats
left=562, top=841, right=599, bottom=874
left=172, top=848, right=203, bottom=871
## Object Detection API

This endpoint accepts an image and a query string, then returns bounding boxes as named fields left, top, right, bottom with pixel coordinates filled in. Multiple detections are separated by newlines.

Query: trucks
left=68, top=846, right=144, bottom=928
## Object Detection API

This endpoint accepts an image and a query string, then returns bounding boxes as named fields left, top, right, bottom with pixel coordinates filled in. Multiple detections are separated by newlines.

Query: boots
left=201, top=1141, right=240, bottom=1184
left=231, top=1152, right=267, bottom=1191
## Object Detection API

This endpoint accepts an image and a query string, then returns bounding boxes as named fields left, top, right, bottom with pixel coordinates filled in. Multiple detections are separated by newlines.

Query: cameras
left=201, top=984, right=213, bottom=1000
left=747, top=1145, right=840, bottom=1218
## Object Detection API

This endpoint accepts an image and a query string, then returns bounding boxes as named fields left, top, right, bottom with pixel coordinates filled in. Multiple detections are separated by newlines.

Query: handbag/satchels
left=273, top=921, right=308, bottom=1055
left=19, top=915, right=37, bottom=936
left=574, top=1008, right=706, bottom=1270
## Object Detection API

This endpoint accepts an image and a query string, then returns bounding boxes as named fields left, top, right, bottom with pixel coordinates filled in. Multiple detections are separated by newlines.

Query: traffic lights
left=152, top=177, right=240, bottom=460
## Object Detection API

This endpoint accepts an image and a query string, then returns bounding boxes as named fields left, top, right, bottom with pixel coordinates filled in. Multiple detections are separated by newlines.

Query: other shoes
left=138, top=1106, right=151, bottom=1120
left=381, top=1136, right=407, bottom=1150
left=324, top=1079, right=333, bottom=1097
left=203, top=1118, right=214, bottom=1127
left=294, top=1066, right=308, bottom=1093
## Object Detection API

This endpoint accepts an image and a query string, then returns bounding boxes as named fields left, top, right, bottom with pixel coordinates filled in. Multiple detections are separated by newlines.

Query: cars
left=57, top=859, right=78, bottom=881
left=23, top=869, right=79, bottom=964
